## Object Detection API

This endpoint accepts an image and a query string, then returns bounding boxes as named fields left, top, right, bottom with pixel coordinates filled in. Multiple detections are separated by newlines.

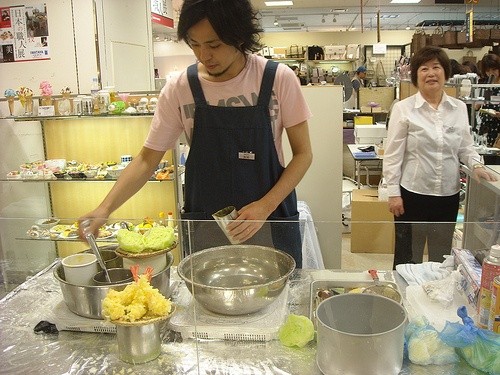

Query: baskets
left=311, top=76, right=333, bottom=83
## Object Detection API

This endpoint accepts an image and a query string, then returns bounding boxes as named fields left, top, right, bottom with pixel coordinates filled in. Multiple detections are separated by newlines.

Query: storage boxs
left=354, top=117, right=373, bottom=125
left=355, top=137, right=381, bottom=144
left=352, top=189, right=428, bottom=256
left=353, top=125, right=386, bottom=137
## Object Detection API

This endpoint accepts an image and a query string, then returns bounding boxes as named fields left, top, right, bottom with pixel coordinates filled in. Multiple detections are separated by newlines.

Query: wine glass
left=118, top=92, right=130, bottom=110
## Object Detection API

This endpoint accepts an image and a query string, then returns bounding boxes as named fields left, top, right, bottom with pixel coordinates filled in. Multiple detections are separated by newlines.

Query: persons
left=382, top=46, right=498, bottom=273
left=462, top=61, right=477, bottom=75
left=2, top=10, right=10, bottom=21
left=476, top=54, right=500, bottom=109
left=351, top=65, right=367, bottom=110
left=452, top=65, right=471, bottom=76
left=77, top=0, right=313, bottom=269
left=290, top=65, right=307, bottom=86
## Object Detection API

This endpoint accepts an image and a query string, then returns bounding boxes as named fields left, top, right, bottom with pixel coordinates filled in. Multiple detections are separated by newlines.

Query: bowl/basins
left=106, top=166, right=127, bottom=177
left=53, top=244, right=174, bottom=320
left=83, top=170, right=97, bottom=178
left=177, top=245, right=296, bottom=316
left=68, top=171, right=86, bottom=178
left=35, top=217, right=60, bottom=229
left=53, top=171, right=67, bottom=178
left=123, top=253, right=166, bottom=277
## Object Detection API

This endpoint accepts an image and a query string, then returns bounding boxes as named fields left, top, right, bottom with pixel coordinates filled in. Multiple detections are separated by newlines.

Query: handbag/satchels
left=308, top=45, right=323, bottom=60
left=286, top=44, right=306, bottom=61
left=354, top=115, right=373, bottom=129
left=376, top=61, right=387, bottom=86
left=324, top=45, right=346, bottom=60
left=413, top=23, right=500, bottom=57
left=313, top=65, right=333, bottom=78
left=401, top=64, right=410, bottom=79
left=399, top=55, right=409, bottom=66
left=347, top=43, right=360, bottom=59
left=364, top=58, right=375, bottom=80
left=440, top=306, right=500, bottom=375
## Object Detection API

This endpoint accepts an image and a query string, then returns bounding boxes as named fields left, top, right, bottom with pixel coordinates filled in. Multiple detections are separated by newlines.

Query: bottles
left=477, top=244, right=500, bottom=334
left=90, top=78, right=100, bottom=97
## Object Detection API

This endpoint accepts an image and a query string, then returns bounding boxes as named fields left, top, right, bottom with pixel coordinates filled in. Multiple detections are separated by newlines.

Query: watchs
left=473, top=164, right=482, bottom=168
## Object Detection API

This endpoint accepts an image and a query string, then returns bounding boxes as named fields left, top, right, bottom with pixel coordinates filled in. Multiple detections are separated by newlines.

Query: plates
left=44, top=159, right=66, bottom=171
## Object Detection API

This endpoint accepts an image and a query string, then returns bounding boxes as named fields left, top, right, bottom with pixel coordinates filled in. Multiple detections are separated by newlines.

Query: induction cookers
left=169, top=281, right=293, bottom=343
left=51, top=277, right=179, bottom=333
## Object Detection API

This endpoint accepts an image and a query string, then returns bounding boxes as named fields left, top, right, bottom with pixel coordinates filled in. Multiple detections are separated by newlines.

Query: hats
left=357, top=66, right=366, bottom=72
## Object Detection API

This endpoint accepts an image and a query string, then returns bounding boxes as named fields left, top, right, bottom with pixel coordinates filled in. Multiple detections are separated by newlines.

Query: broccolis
left=407, top=329, right=457, bottom=364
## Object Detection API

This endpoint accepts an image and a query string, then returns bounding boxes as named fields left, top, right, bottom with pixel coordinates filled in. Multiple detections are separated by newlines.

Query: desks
left=347, top=144, right=384, bottom=189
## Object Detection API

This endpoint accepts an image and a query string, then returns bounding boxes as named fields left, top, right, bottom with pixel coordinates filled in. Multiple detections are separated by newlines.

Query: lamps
left=274, top=20, right=278, bottom=25
left=321, top=13, right=336, bottom=22
left=153, top=7, right=182, bottom=43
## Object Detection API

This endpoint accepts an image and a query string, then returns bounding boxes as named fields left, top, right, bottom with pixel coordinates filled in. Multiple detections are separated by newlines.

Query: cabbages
left=117, top=226, right=178, bottom=253
left=279, top=314, right=315, bottom=346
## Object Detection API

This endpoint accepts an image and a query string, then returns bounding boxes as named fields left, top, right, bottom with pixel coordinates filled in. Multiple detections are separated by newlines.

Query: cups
left=72, top=97, right=93, bottom=115
left=121, top=155, right=132, bottom=167
left=61, top=253, right=98, bottom=285
left=93, top=268, right=133, bottom=286
left=116, top=322, right=161, bottom=363
left=211, top=206, right=242, bottom=245
left=315, top=293, right=408, bottom=375
left=83, top=249, right=119, bottom=271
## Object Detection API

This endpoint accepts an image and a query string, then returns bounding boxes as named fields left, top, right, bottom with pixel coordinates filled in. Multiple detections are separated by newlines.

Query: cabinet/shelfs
left=461, top=165, right=500, bottom=254
left=0, top=90, right=181, bottom=293
left=265, top=42, right=500, bottom=160
